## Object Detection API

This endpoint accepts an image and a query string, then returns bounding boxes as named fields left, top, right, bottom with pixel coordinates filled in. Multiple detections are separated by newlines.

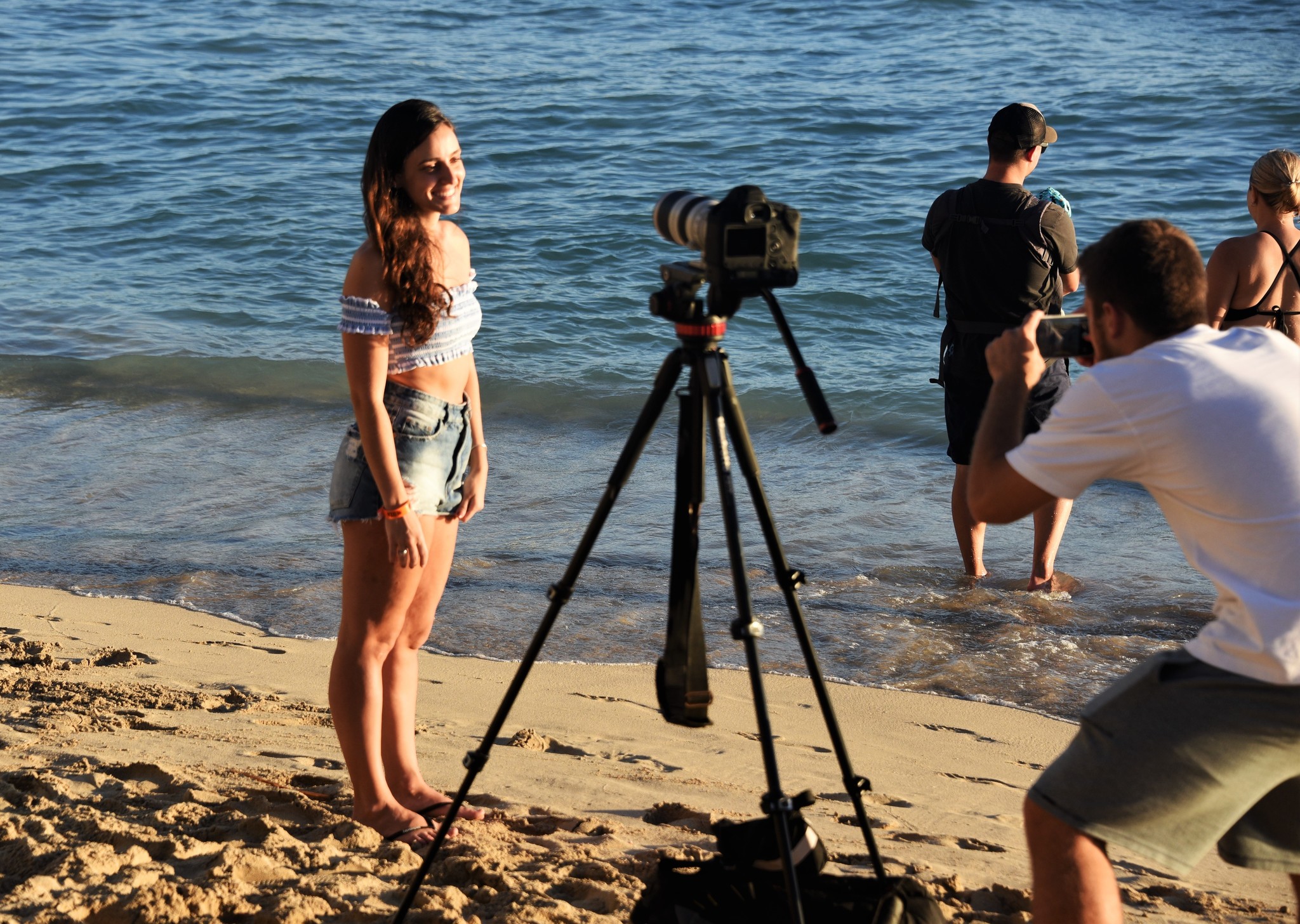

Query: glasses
left=1025, top=142, right=1049, bottom=154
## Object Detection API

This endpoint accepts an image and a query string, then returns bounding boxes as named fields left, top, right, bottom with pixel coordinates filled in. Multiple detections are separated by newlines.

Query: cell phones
left=1026, top=312, right=1094, bottom=358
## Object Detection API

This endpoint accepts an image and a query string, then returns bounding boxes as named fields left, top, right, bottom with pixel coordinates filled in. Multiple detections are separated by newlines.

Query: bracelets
left=376, top=499, right=412, bottom=522
left=470, top=443, right=489, bottom=452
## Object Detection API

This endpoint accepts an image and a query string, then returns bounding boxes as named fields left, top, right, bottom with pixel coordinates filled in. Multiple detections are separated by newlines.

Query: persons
left=963, top=215, right=1300, bottom=924
left=321, top=97, right=490, bottom=851
left=919, top=99, right=1082, bottom=602
left=1202, top=147, right=1300, bottom=348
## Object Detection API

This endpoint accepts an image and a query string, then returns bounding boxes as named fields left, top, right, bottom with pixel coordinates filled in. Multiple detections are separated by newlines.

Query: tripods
left=390, top=293, right=910, bottom=924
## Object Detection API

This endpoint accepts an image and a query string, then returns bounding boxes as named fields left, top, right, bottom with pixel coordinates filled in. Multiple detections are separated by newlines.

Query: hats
left=988, top=103, right=1057, bottom=150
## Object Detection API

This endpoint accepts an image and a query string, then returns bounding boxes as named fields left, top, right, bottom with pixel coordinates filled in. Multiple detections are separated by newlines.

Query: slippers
left=416, top=800, right=485, bottom=822
left=386, top=814, right=449, bottom=851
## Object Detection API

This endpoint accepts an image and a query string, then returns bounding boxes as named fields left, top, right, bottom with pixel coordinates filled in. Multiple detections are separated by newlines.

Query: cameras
left=653, top=186, right=804, bottom=292
